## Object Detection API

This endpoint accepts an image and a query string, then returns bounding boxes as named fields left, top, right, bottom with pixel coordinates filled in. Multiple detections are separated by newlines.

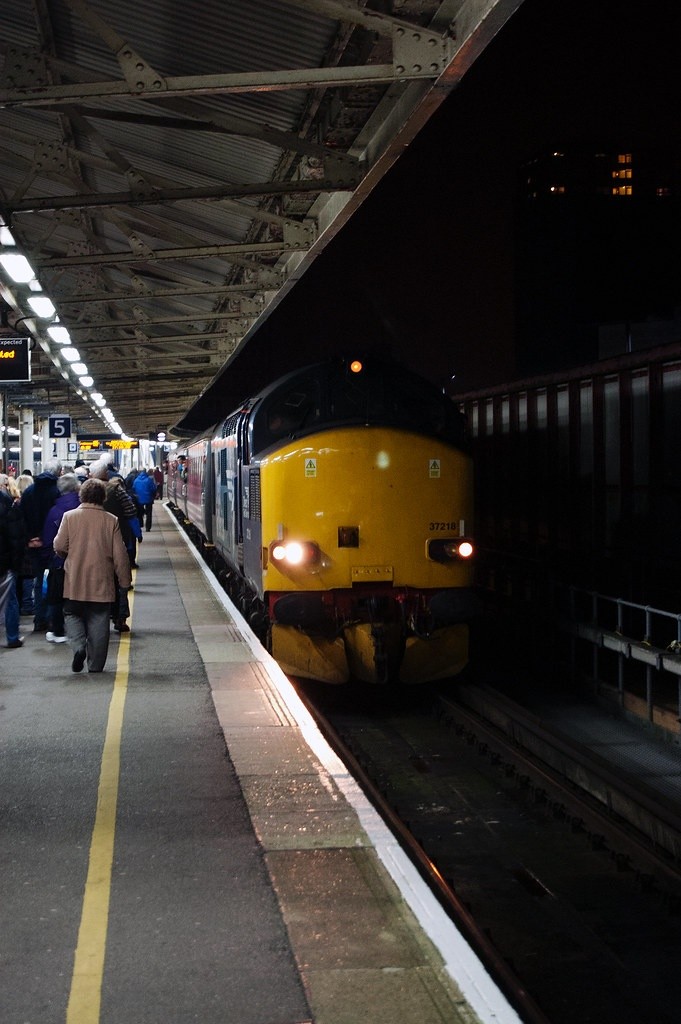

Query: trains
left=168, top=351, right=481, bottom=684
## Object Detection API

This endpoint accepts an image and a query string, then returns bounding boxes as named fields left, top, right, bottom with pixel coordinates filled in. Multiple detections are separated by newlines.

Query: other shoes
left=88, top=669, right=103, bottom=673
left=131, top=564, right=139, bottom=569
left=156, top=498, right=159, bottom=500
left=7, top=639, right=23, bottom=648
left=146, top=528, right=150, bottom=532
left=72, top=646, right=86, bottom=672
left=160, top=497, right=162, bottom=500
left=114, top=624, right=130, bottom=632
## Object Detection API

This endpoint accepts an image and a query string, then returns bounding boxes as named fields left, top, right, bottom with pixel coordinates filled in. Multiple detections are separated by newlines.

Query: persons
left=53, top=478, right=131, bottom=673
left=171, top=455, right=187, bottom=479
left=162, top=460, right=168, bottom=475
left=0, top=462, right=164, bottom=648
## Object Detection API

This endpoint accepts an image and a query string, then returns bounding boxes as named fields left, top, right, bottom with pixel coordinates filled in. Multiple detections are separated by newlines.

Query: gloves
left=138, top=536, right=142, bottom=543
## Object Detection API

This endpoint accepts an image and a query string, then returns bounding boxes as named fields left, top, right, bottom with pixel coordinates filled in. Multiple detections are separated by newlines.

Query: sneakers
left=46, top=632, right=68, bottom=643
